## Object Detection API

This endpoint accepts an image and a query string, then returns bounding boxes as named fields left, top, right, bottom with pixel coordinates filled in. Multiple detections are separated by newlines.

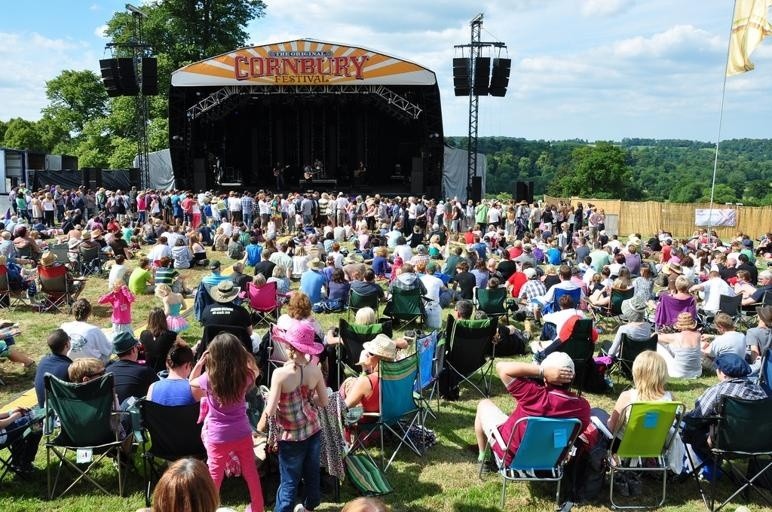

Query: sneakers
left=476, top=455, right=493, bottom=474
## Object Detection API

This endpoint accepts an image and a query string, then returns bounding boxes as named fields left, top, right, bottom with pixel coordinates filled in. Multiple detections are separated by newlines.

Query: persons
left=358, top=161, right=367, bottom=185
left=0, top=182, right=772, bottom=512
left=304, top=164, right=313, bottom=188
left=313, top=158, right=324, bottom=179
left=274, top=161, right=285, bottom=190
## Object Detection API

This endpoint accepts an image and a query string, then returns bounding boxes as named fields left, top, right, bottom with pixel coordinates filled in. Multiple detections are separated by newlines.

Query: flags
left=727, top=1, right=772, bottom=78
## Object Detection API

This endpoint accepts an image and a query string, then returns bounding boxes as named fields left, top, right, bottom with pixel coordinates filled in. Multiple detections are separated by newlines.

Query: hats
left=362, top=332, right=397, bottom=360
left=672, top=311, right=699, bottom=330
left=208, top=260, right=222, bottom=270
left=754, top=304, right=772, bottom=326
left=540, top=352, right=575, bottom=379
left=310, top=224, right=538, bottom=280
left=209, top=280, right=242, bottom=304
left=666, top=262, right=683, bottom=273
left=711, top=352, right=754, bottom=378
left=111, top=331, right=138, bottom=353
left=5, top=180, right=162, bottom=265
left=620, top=295, right=649, bottom=321
left=271, top=323, right=324, bottom=356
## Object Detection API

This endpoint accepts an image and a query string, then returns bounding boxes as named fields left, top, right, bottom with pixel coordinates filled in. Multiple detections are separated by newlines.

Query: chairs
left=0, top=412, right=46, bottom=485
left=42, top=371, right=136, bottom=502
left=0, top=231, right=103, bottom=316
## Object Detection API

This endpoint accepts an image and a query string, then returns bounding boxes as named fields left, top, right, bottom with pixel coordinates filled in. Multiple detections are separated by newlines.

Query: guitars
left=304, top=171, right=320, bottom=180
left=273, top=165, right=290, bottom=176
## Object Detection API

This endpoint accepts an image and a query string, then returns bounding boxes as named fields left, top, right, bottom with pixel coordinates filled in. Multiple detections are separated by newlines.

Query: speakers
left=177, top=158, right=207, bottom=194
left=99, top=57, right=158, bottom=98
left=471, top=176, right=482, bottom=207
left=80, top=167, right=89, bottom=188
left=90, top=168, right=102, bottom=190
left=525, top=181, right=534, bottom=207
left=390, top=157, right=424, bottom=194
left=453, top=57, right=510, bottom=97
left=512, top=181, right=525, bottom=205
